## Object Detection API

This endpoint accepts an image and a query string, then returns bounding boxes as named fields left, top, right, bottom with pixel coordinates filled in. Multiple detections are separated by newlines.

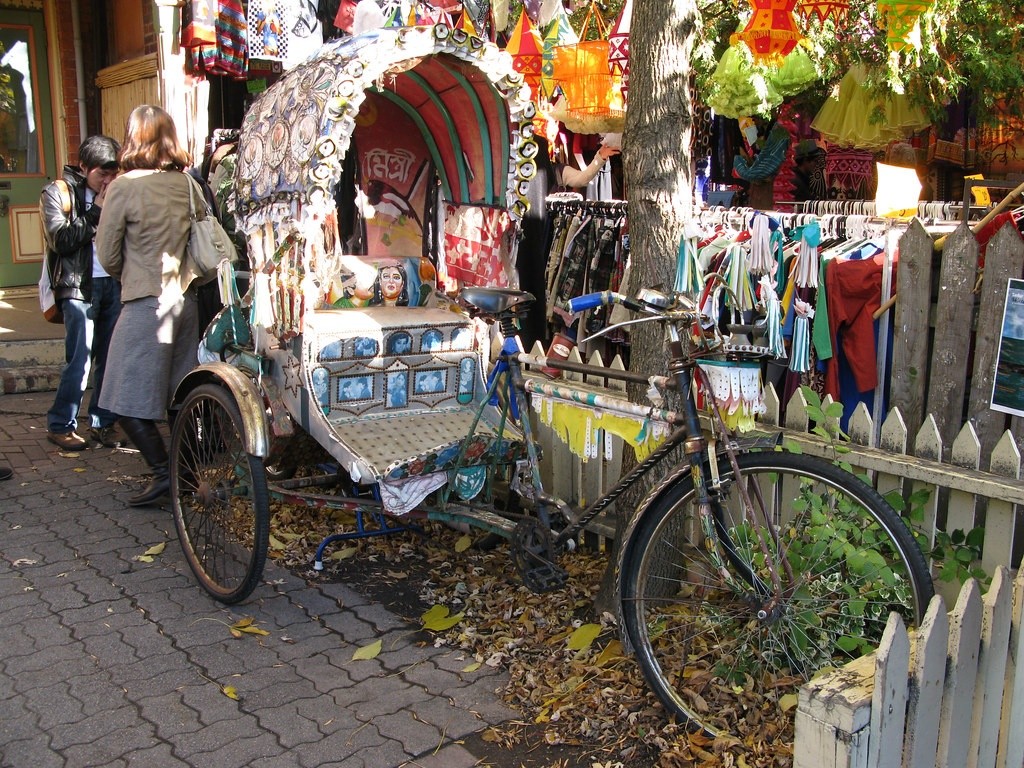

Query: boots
left=167, top=409, right=201, bottom=496
left=118, top=414, right=182, bottom=506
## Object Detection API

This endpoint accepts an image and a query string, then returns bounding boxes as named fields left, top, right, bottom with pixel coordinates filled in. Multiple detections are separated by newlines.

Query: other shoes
left=0, top=466, right=13, bottom=480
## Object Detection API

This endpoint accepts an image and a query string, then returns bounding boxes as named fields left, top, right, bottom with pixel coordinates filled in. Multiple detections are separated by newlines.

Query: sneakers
left=47, top=430, right=87, bottom=451
left=91, top=425, right=127, bottom=448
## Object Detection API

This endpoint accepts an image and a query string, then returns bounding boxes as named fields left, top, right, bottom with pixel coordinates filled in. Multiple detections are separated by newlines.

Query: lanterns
left=731, top=0, right=802, bottom=66
left=504, top=0, right=633, bottom=135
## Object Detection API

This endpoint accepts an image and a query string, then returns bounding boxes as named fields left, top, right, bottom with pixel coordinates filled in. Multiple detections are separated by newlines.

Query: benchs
left=281, top=253, right=487, bottom=418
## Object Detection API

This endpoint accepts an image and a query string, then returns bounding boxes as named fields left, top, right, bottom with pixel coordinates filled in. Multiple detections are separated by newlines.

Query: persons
left=95, top=106, right=216, bottom=506
left=39, top=135, right=127, bottom=449
left=186, top=166, right=228, bottom=459
left=547, top=142, right=620, bottom=197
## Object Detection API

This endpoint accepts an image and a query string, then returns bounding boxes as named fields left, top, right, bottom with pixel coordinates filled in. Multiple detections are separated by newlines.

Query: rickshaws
left=162, top=24, right=936, bottom=755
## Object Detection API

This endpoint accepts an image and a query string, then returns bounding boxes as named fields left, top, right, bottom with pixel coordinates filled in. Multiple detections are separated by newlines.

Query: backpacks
left=39, top=179, right=75, bottom=323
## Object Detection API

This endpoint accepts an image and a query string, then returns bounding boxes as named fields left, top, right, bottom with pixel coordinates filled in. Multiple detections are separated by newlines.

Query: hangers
left=547, top=194, right=1024, bottom=268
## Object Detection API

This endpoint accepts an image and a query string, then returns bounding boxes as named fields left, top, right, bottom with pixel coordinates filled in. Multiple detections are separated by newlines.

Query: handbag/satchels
left=183, top=171, right=238, bottom=287
